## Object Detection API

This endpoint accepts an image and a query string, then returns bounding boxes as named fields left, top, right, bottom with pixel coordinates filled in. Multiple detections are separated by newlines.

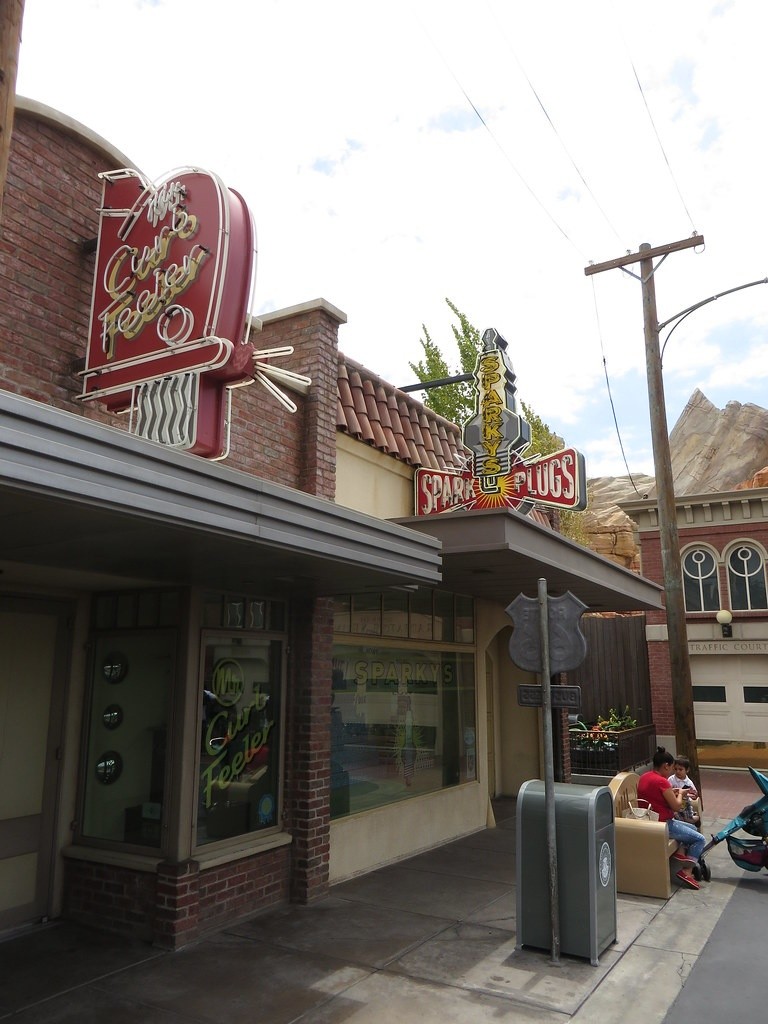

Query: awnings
left=381, top=507, right=665, bottom=612
left=1, top=388, right=443, bottom=598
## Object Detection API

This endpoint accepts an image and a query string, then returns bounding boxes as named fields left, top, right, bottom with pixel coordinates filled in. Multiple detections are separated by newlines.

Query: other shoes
left=676, top=871, right=700, bottom=889
left=673, top=854, right=696, bottom=866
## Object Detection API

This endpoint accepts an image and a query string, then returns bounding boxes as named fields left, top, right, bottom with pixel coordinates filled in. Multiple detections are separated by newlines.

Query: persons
left=667, top=755, right=701, bottom=828
left=637, top=745, right=705, bottom=889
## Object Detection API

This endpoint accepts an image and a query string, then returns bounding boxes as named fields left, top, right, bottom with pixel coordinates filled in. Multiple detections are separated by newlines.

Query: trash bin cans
left=513, top=780, right=619, bottom=966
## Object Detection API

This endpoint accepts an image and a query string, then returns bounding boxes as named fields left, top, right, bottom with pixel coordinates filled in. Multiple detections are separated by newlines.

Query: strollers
left=690, top=765, right=768, bottom=882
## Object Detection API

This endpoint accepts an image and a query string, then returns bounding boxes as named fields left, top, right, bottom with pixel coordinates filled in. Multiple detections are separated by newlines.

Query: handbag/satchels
left=622, top=799, right=659, bottom=821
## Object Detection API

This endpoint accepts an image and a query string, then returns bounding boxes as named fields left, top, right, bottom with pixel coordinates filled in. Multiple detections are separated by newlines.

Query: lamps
left=715, top=609, right=733, bottom=638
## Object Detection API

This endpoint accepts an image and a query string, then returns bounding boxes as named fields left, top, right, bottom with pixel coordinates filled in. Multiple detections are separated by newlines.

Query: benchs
left=609, top=771, right=704, bottom=898
